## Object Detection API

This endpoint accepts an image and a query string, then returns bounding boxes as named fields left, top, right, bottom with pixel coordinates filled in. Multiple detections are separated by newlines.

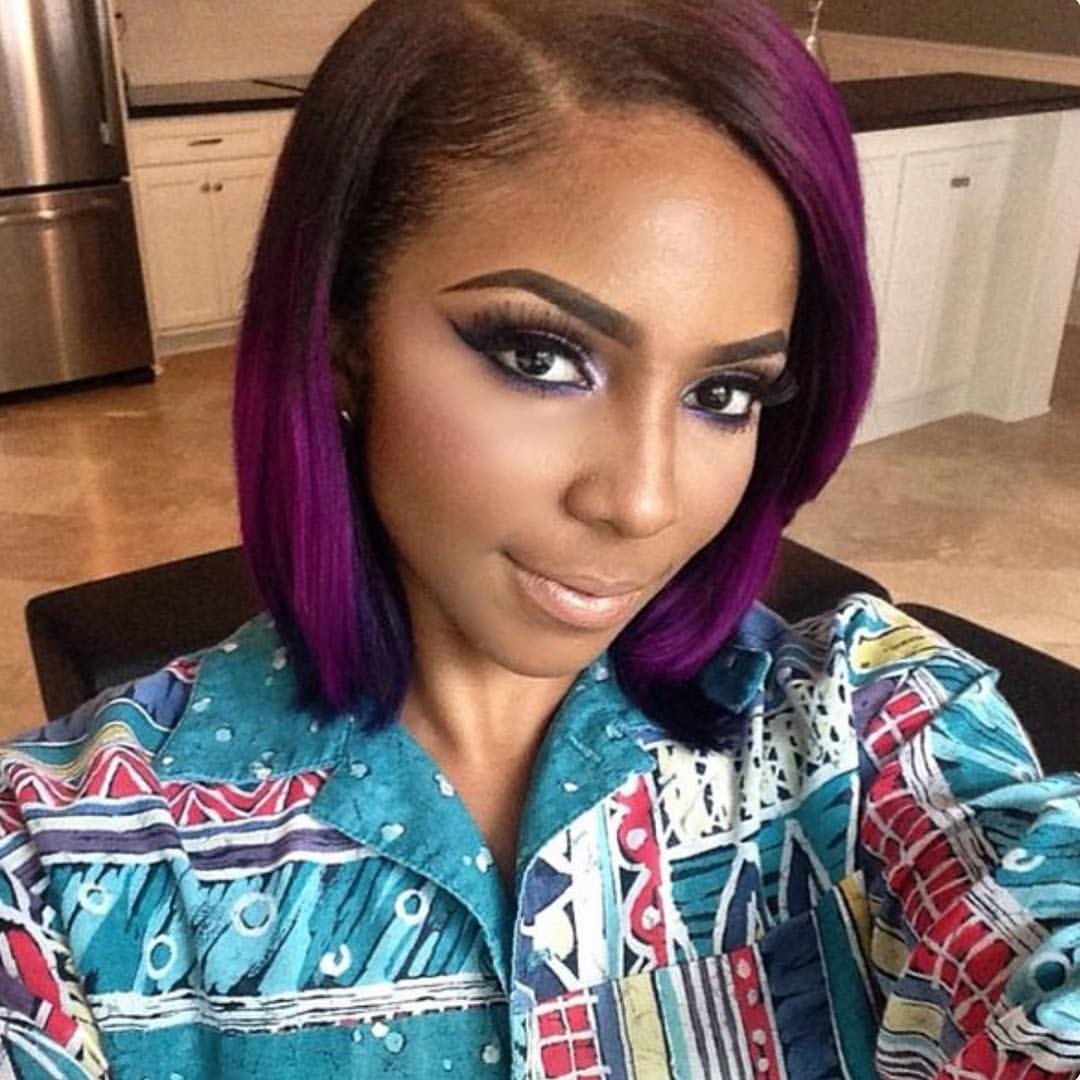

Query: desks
left=897, top=597, right=1079, bottom=780
left=23, top=504, right=890, bottom=732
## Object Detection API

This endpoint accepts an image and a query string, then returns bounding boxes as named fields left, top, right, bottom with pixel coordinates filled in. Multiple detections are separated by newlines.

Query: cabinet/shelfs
left=131, top=109, right=313, bottom=379
left=879, top=130, right=1027, bottom=441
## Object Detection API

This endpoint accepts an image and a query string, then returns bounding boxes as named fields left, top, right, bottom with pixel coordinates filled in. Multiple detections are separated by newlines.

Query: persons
left=0, top=6, right=1080, bottom=1080
